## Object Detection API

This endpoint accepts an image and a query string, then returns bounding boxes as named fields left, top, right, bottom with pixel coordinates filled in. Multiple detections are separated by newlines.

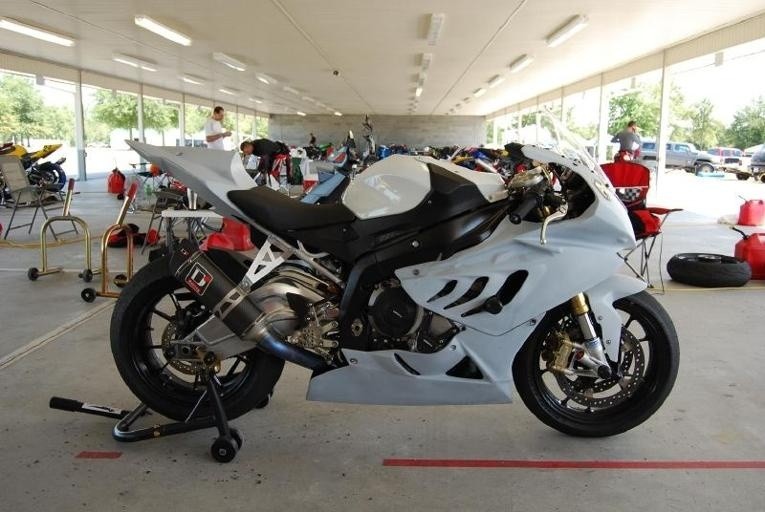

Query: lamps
left=408, top=11, right=586, bottom=120
left=215, top=51, right=248, bottom=72
left=182, top=75, right=207, bottom=86
left=217, top=73, right=345, bottom=120
left=112, top=53, right=156, bottom=71
left=134, top=16, right=193, bottom=46
left=1, top=17, right=75, bottom=47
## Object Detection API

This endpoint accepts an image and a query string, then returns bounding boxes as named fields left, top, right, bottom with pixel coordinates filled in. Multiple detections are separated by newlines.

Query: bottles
left=144, top=185, right=152, bottom=205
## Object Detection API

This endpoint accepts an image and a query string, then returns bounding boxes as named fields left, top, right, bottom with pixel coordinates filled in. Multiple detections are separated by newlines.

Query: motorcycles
left=0, top=142, right=66, bottom=192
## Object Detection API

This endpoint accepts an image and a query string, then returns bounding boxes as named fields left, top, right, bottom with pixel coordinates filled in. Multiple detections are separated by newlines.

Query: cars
left=640, top=138, right=765, bottom=184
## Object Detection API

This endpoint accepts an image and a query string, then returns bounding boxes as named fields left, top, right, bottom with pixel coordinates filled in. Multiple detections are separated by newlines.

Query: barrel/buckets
left=737, top=195, right=765, bottom=227
left=107, top=172, right=124, bottom=193
left=731, top=227, right=765, bottom=281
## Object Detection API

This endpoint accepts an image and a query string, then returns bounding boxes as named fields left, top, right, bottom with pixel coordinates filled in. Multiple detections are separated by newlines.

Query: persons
left=610, top=121, right=643, bottom=156
left=309, top=133, right=317, bottom=147
left=205, top=105, right=232, bottom=151
left=240, top=138, right=291, bottom=184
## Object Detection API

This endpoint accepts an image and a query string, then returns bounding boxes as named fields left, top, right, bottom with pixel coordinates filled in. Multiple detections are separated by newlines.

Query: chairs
left=598, top=165, right=682, bottom=294
left=1, top=153, right=80, bottom=242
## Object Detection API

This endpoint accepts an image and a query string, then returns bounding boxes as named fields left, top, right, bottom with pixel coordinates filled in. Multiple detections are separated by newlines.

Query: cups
left=186, top=188, right=197, bottom=210
